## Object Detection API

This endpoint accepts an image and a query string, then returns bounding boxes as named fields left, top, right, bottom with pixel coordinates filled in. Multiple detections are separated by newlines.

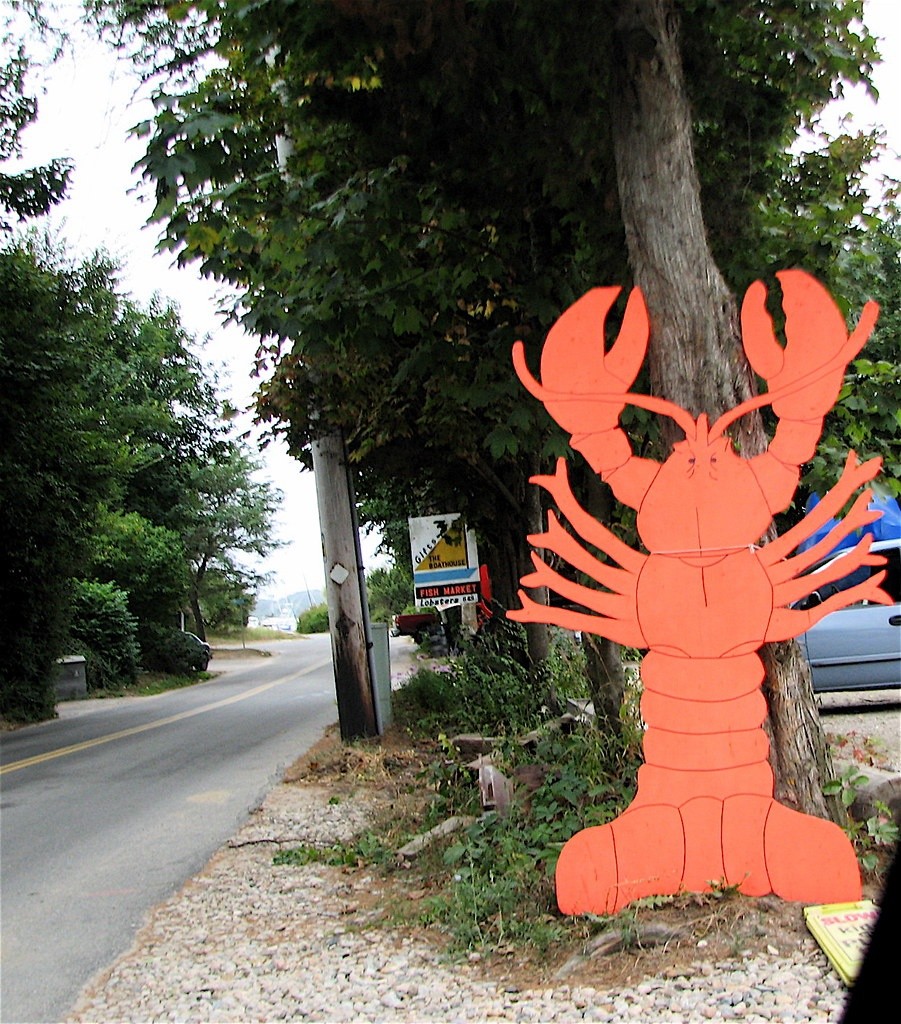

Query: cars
left=789, top=539, right=901, bottom=694
left=183, top=632, right=211, bottom=671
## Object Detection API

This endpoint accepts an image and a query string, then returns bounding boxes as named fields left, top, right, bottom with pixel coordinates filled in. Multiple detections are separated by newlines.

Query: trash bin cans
left=52, top=653, right=88, bottom=702
